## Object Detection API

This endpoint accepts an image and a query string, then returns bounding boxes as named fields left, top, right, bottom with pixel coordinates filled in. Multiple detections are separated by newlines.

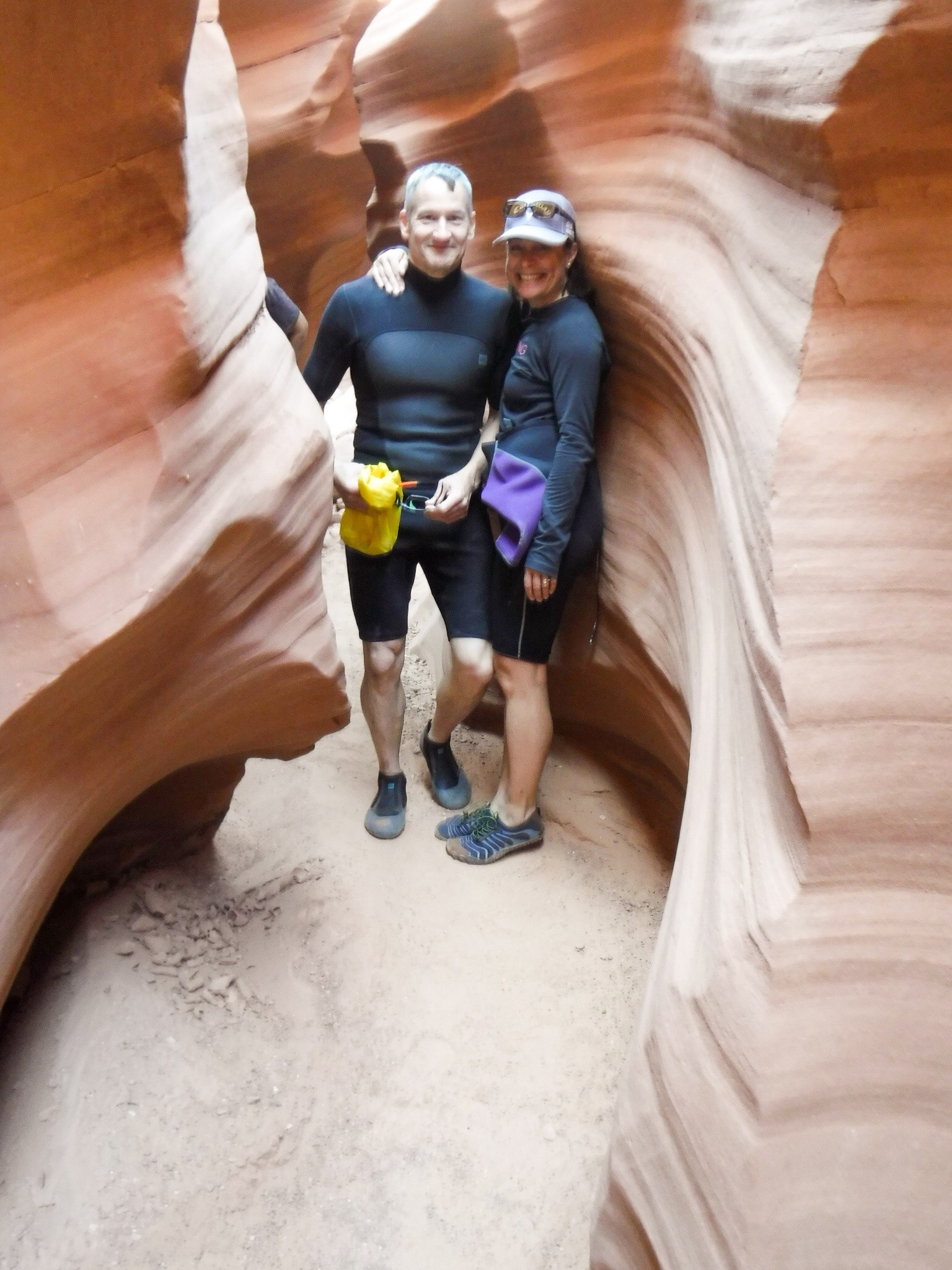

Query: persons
left=264, top=275, right=308, bottom=356
left=367, top=189, right=610, bottom=865
left=300, top=161, right=523, bottom=840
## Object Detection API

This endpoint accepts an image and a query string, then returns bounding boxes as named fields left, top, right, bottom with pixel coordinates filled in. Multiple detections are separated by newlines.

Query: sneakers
left=365, top=773, right=409, bottom=839
left=419, top=720, right=473, bottom=809
left=434, top=803, right=545, bottom=865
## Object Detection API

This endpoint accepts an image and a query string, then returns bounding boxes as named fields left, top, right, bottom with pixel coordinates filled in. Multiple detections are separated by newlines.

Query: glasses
left=393, top=482, right=444, bottom=511
left=502, top=197, right=577, bottom=238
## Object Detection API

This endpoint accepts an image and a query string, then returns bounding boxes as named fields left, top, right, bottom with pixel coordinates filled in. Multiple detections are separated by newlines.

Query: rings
left=542, top=578, right=550, bottom=584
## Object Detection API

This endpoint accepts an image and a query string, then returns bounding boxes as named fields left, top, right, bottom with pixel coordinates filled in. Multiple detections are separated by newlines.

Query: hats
left=490, top=190, right=579, bottom=248
